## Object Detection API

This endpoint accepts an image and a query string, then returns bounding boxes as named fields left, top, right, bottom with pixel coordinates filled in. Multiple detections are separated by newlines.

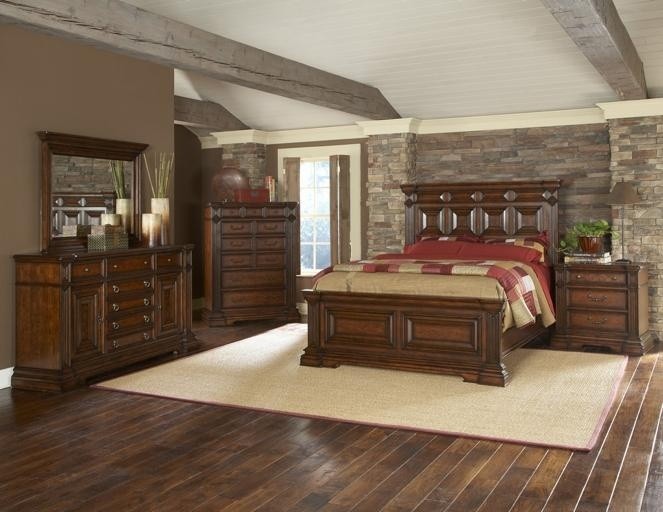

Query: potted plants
left=559, top=217, right=623, bottom=256
left=109, top=158, right=133, bottom=234
left=140, top=150, right=177, bottom=246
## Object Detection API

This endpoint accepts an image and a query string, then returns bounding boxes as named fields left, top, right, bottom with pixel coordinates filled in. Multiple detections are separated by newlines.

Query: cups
left=140, top=212, right=164, bottom=248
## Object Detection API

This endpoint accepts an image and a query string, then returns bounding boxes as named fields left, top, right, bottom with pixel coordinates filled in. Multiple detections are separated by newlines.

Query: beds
left=299, top=179, right=562, bottom=388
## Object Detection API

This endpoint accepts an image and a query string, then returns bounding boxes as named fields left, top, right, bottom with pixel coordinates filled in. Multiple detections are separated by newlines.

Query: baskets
left=87, top=225, right=127, bottom=253
left=62, top=224, right=90, bottom=235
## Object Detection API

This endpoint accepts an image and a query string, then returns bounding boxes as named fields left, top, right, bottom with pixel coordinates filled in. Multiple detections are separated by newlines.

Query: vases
left=142, top=213, right=163, bottom=246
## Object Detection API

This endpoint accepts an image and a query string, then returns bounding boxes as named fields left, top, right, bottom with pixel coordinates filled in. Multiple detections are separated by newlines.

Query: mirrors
left=35, top=129, right=149, bottom=255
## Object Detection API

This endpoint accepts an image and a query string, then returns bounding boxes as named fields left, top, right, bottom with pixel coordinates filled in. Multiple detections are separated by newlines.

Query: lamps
left=604, top=182, right=640, bottom=264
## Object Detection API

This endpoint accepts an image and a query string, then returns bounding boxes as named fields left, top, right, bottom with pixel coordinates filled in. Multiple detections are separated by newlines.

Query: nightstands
left=549, top=262, right=658, bottom=358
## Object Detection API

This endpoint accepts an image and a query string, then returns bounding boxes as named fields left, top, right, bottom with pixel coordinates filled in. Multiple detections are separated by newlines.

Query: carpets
left=88, top=321, right=630, bottom=452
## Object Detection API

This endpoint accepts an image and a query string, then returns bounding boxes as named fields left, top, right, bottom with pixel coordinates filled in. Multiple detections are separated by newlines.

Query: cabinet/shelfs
left=200, top=201, right=303, bottom=326
left=12, top=241, right=194, bottom=394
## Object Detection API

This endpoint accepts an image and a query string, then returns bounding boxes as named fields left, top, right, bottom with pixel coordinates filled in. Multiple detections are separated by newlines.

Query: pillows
left=404, top=229, right=550, bottom=263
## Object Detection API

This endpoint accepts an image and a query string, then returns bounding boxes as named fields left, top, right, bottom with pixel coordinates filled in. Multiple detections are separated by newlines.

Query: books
left=264, top=175, right=279, bottom=203
left=567, top=250, right=610, bottom=259
left=563, top=256, right=612, bottom=265
